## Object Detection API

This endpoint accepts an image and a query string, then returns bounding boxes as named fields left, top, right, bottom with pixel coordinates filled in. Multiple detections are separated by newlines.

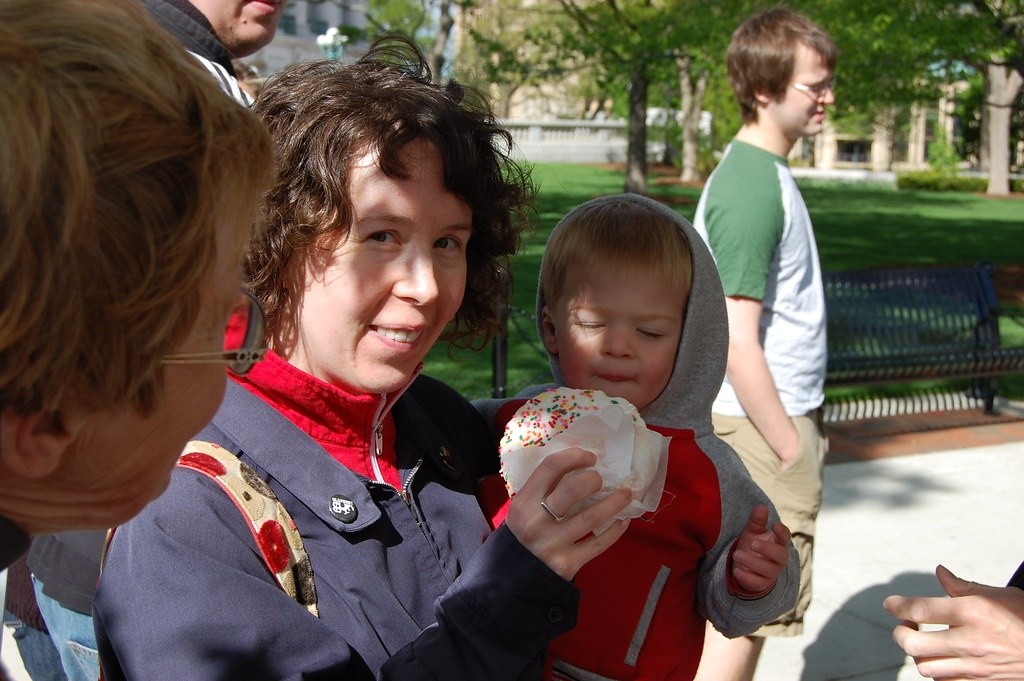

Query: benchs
left=815, top=257, right=1024, bottom=439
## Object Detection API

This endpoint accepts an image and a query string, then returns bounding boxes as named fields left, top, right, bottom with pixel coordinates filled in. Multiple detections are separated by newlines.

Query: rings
left=541, top=497, right=567, bottom=522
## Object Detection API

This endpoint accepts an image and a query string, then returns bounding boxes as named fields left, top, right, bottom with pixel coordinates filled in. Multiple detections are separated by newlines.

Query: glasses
left=789, top=75, right=836, bottom=100
left=159, top=287, right=269, bottom=376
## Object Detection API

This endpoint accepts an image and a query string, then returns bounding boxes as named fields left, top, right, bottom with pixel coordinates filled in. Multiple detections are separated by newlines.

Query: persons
left=92, top=41, right=631, bottom=681
left=0, top=2, right=276, bottom=570
left=881, top=556, right=1024, bottom=681
left=692, top=9, right=837, bottom=681
left=472, top=193, right=800, bottom=681
left=0, top=0, right=290, bottom=679
